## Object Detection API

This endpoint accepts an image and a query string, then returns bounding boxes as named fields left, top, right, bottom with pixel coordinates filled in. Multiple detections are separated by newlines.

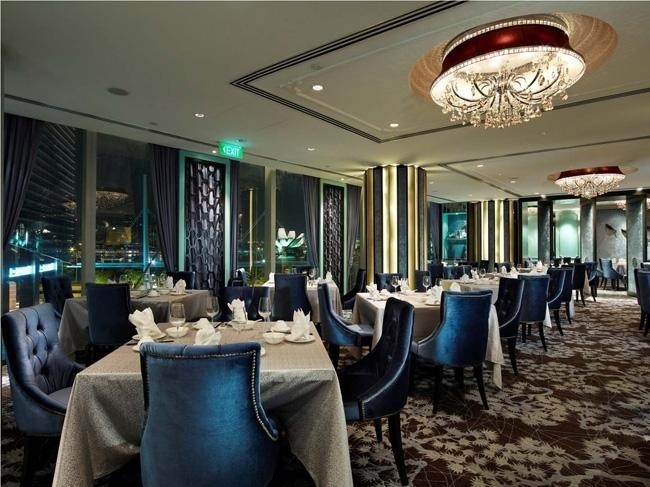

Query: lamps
left=553, top=165, right=626, bottom=200
left=429, top=14, right=587, bottom=129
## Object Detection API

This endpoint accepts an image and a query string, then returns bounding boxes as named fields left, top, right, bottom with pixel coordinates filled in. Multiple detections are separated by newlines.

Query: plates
left=170, top=292, right=186, bottom=296
left=132, top=332, right=167, bottom=340
left=284, top=334, right=315, bottom=343
left=147, top=295, right=160, bottom=298
left=425, top=302, right=440, bottom=306
left=132, top=345, right=140, bottom=352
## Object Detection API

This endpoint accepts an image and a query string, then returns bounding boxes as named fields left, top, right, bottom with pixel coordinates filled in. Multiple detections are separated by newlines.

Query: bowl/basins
left=149, top=291, right=158, bottom=295
left=272, top=320, right=290, bottom=332
left=137, top=336, right=155, bottom=349
left=262, top=332, right=285, bottom=344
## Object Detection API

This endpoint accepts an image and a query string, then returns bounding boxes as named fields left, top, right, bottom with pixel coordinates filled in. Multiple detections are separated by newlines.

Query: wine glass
left=391, top=260, right=556, bottom=294
left=168, top=297, right=271, bottom=343
left=119, top=273, right=168, bottom=290
left=285, top=268, right=319, bottom=281
